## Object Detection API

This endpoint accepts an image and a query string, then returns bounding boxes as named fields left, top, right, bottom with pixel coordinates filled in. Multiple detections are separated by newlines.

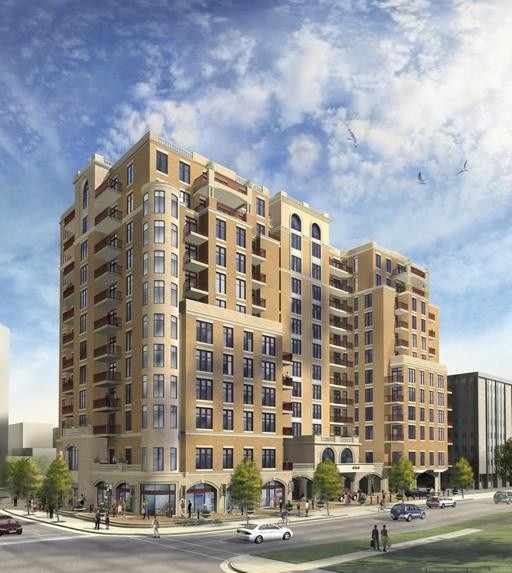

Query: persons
left=152, top=515, right=160, bottom=538
left=372, top=525, right=381, bottom=551
left=380, top=495, right=386, bottom=511
left=305, top=500, right=309, bottom=516
left=141, top=502, right=146, bottom=519
left=381, top=525, right=390, bottom=552
left=226, top=503, right=233, bottom=514
left=117, top=496, right=124, bottom=514
left=280, top=510, right=289, bottom=519
left=111, top=501, right=117, bottom=518
left=168, top=502, right=174, bottom=518
left=105, top=510, right=110, bottom=529
left=164, top=503, right=168, bottom=516
left=145, top=501, right=150, bottom=520
left=188, top=500, right=192, bottom=518
left=181, top=500, right=185, bottom=519
left=79, top=493, right=87, bottom=507
left=94, top=510, right=100, bottom=530
left=429, top=486, right=435, bottom=496
left=297, top=502, right=301, bottom=513
